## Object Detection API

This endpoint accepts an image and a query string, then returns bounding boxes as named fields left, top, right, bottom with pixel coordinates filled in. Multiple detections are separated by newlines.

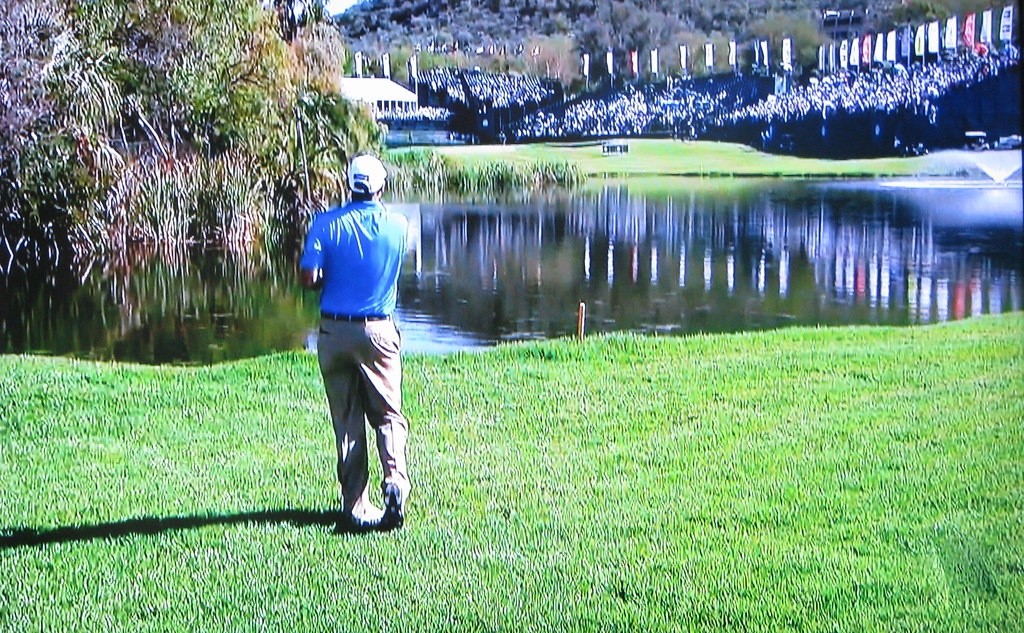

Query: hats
left=349, top=154, right=387, bottom=193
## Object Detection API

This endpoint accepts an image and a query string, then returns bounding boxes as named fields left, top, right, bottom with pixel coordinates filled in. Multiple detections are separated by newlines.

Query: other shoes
left=383, top=484, right=403, bottom=529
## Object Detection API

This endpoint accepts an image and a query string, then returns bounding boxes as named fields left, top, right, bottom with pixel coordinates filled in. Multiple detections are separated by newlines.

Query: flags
left=705, top=44, right=713, bottom=66
left=915, top=25, right=924, bottom=56
left=980, top=10, right=990, bottom=42
left=928, top=22, right=938, bottom=52
left=728, top=42, right=736, bottom=66
left=631, top=51, right=638, bottom=73
left=680, top=46, right=686, bottom=69
left=783, top=39, right=791, bottom=64
left=901, top=28, right=908, bottom=55
left=651, top=50, right=657, bottom=73
left=887, top=31, right=895, bottom=60
left=945, top=18, right=956, bottom=48
left=963, top=15, right=974, bottom=46
left=606, top=53, right=613, bottom=74
left=850, top=39, right=858, bottom=65
left=840, top=40, right=847, bottom=67
left=874, top=33, right=883, bottom=62
left=583, top=53, right=589, bottom=76
left=862, top=35, right=870, bottom=63
left=1000, top=6, right=1012, bottom=41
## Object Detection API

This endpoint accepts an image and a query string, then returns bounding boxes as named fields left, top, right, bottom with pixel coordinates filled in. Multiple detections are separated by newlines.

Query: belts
left=320, top=310, right=387, bottom=322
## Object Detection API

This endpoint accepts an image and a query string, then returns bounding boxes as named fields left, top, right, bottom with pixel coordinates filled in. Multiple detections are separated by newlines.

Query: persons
left=498, top=131, right=506, bottom=145
left=298, top=155, right=412, bottom=532
left=430, top=65, right=555, bottom=108
left=374, top=106, right=454, bottom=120
left=515, top=44, right=1020, bottom=137
